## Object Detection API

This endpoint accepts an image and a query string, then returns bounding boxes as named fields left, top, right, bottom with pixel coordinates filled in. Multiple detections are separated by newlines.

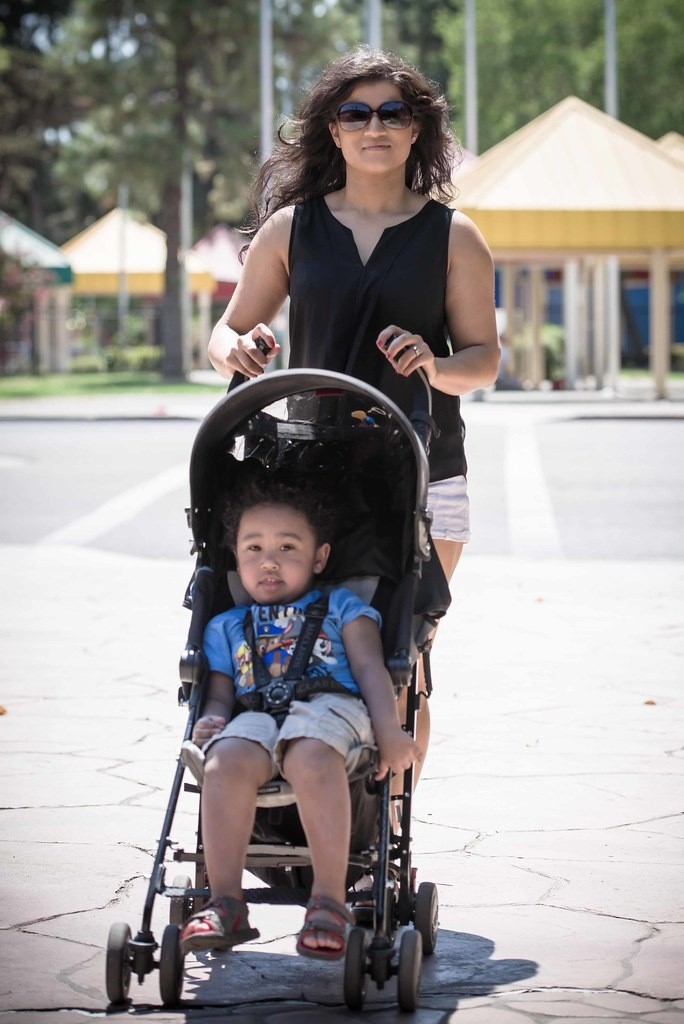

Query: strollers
left=104, top=336, right=439, bottom=1013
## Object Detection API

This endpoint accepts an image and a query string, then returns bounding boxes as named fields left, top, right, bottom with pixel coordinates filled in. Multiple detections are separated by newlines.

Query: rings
left=411, top=345, right=420, bottom=358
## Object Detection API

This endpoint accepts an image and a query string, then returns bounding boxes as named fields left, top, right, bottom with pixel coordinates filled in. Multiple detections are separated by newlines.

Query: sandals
left=179, top=895, right=260, bottom=951
left=296, top=894, right=356, bottom=961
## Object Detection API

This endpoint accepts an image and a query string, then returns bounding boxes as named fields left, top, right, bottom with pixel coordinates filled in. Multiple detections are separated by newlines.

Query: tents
left=0, top=96, right=684, bottom=391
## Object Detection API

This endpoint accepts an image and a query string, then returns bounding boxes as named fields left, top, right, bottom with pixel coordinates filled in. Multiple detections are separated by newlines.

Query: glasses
left=331, top=101, right=416, bottom=131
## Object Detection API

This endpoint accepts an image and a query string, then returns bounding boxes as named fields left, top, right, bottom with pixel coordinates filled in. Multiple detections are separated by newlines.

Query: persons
left=206, top=48, right=495, bottom=826
left=179, top=481, right=426, bottom=960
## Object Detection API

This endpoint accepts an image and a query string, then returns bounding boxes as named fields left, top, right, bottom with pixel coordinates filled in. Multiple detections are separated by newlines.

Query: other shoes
left=350, top=886, right=377, bottom=925
